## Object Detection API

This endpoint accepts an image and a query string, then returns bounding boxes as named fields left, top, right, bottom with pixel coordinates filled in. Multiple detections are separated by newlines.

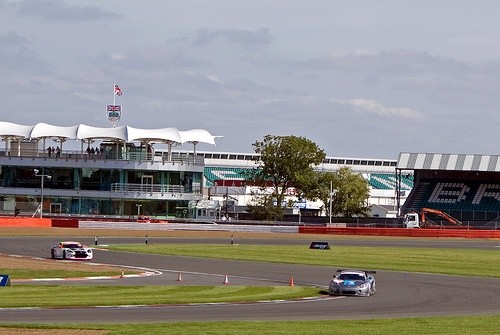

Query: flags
left=114, top=84, right=122, bottom=96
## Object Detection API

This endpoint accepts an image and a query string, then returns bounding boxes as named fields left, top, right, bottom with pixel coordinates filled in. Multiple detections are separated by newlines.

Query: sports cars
left=328, top=267, right=377, bottom=297
left=50, top=241, right=94, bottom=261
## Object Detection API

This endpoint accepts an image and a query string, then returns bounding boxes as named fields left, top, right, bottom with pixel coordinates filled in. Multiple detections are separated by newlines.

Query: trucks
left=401, top=211, right=421, bottom=228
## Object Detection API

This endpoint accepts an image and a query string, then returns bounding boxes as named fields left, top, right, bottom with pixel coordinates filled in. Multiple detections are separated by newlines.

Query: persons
left=86, top=147, right=103, bottom=155
left=47, top=146, right=61, bottom=158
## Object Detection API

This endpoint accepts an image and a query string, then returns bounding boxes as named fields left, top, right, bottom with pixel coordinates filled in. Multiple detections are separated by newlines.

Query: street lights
left=33, top=169, right=52, bottom=219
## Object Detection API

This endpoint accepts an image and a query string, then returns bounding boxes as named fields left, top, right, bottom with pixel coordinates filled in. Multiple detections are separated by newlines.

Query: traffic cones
left=222, top=273, right=230, bottom=285
left=119, top=269, right=127, bottom=279
left=175, top=272, right=183, bottom=282
left=288, top=275, right=295, bottom=286
